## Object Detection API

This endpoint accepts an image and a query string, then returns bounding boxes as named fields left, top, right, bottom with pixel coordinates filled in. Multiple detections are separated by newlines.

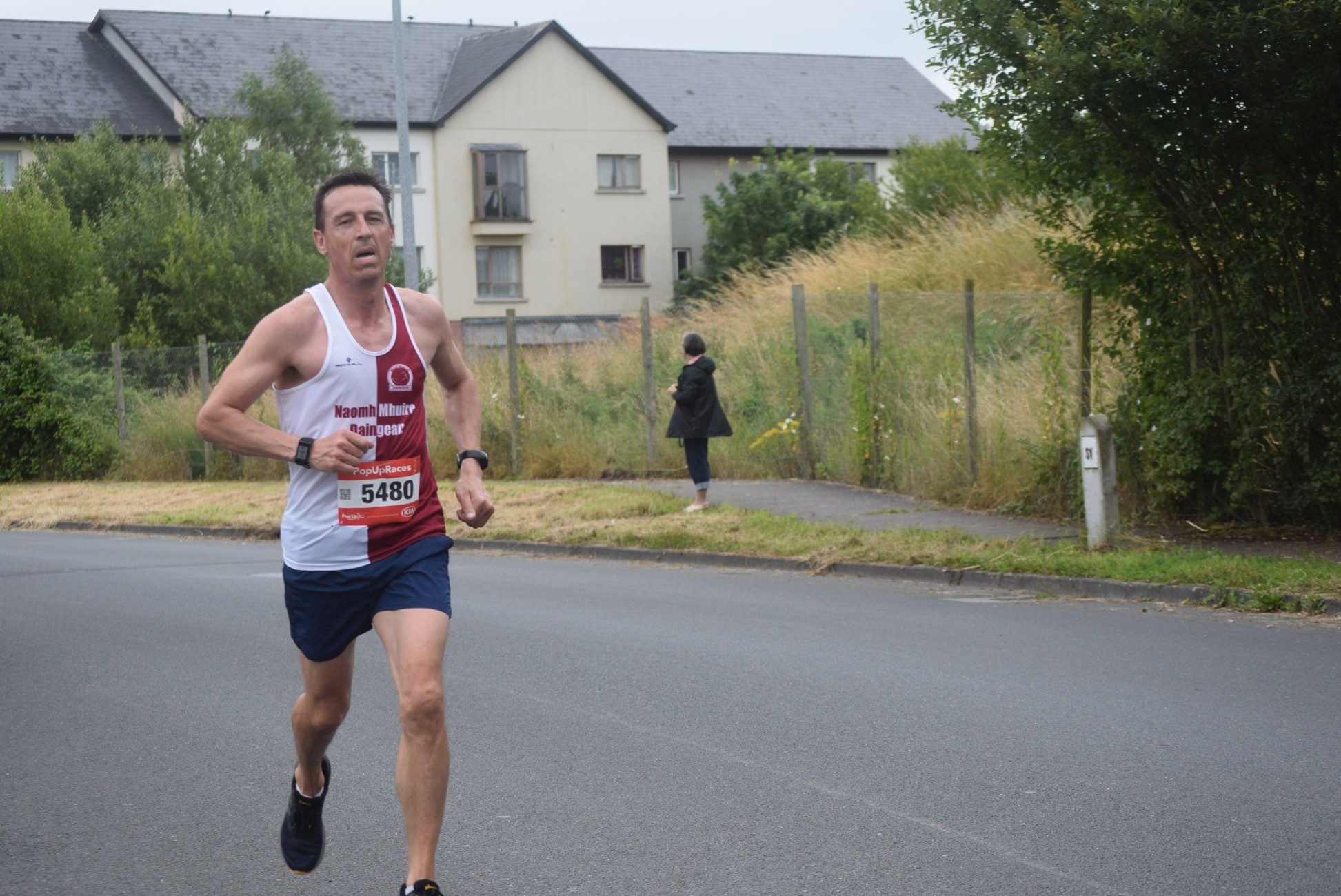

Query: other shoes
left=683, top=502, right=709, bottom=513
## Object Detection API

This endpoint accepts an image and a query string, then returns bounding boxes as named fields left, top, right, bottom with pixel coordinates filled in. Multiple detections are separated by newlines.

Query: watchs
left=456, top=449, right=488, bottom=470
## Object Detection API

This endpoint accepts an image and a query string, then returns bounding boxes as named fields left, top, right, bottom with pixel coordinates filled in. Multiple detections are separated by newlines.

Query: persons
left=665, top=333, right=733, bottom=513
left=195, top=172, right=495, bottom=896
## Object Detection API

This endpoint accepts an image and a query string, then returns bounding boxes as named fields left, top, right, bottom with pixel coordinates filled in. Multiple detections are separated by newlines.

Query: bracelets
left=294, top=437, right=315, bottom=468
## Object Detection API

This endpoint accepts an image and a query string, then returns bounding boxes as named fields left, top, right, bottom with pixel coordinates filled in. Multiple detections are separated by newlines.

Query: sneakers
left=280, top=754, right=331, bottom=874
left=399, top=879, right=445, bottom=896
